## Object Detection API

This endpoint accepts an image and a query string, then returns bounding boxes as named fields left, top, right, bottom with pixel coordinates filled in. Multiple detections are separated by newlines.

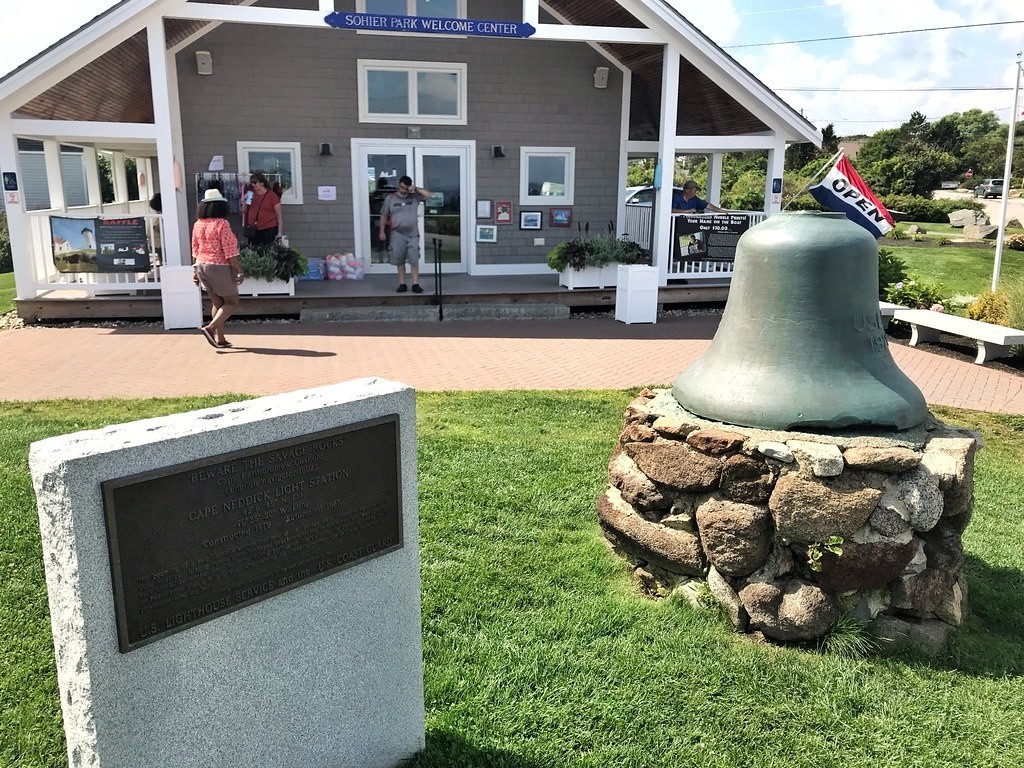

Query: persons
left=671, top=180, right=728, bottom=260
left=147, top=191, right=164, bottom=264
left=191, top=188, right=244, bottom=349
left=687, top=234, right=705, bottom=255
left=246, top=173, right=283, bottom=255
left=379, top=175, right=433, bottom=293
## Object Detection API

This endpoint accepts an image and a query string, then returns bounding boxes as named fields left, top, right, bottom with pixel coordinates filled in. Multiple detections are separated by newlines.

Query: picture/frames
left=549, top=209, right=572, bottom=226
left=520, top=211, right=542, bottom=230
left=494, top=201, right=513, bottom=224
left=476, top=225, right=498, bottom=242
left=476, top=200, right=491, bottom=218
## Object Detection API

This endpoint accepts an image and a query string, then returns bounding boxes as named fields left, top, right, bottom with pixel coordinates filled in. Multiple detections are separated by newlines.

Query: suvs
left=973, top=178, right=1004, bottom=199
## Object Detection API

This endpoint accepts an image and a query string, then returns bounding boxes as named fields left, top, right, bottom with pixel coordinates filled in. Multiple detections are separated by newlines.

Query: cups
left=246, top=191, right=254, bottom=205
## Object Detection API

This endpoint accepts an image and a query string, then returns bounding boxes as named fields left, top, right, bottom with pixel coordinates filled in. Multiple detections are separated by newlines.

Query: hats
left=683, top=180, right=702, bottom=193
left=201, top=188, right=227, bottom=202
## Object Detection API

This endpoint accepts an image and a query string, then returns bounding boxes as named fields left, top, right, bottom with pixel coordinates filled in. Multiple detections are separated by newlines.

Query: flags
left=809, top=152, right=897, bottom=239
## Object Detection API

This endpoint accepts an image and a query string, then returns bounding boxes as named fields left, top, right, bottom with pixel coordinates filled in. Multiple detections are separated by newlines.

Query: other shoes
left=201, top=325, right=218, bottom=348
left=397, top=284, right=408, bottom=293
left=412, top=284, right=423, bottom=293
left=216, top=339, right=233, bottom=348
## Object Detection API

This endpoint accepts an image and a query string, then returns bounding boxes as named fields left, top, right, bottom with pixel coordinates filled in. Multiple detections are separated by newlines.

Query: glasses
left=250, top=180, right=260, bottom=185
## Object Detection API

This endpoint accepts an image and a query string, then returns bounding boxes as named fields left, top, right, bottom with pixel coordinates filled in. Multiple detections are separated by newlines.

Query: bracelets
left=237, top=273, right=244, bottom=277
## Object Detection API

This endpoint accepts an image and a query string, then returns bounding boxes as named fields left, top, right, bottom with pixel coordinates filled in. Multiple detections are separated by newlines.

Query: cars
left=624, top=184, right=722, bottom=213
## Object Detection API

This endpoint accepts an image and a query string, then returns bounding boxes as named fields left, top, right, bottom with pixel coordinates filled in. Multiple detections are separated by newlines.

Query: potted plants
left=238, top=240, right=308, bottom=297
left=547, top=219, right=649, bottom=290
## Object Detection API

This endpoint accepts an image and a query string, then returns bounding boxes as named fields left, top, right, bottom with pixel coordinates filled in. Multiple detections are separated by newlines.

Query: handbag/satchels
left=242, top=225, right=257, bottom=238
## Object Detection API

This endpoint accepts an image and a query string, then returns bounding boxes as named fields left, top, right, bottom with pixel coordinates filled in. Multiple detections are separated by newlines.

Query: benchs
left=894, top=309, right=1024, bottom=365
left=879, top=301, right=910, bottom=328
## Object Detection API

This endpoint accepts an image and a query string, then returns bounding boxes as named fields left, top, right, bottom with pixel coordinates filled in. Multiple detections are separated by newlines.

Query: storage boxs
left=324, top=257, right=368, bottom=281
left=298, top=258, right=326, bottom=281
left=92, top=272, right=136, bottom=296
